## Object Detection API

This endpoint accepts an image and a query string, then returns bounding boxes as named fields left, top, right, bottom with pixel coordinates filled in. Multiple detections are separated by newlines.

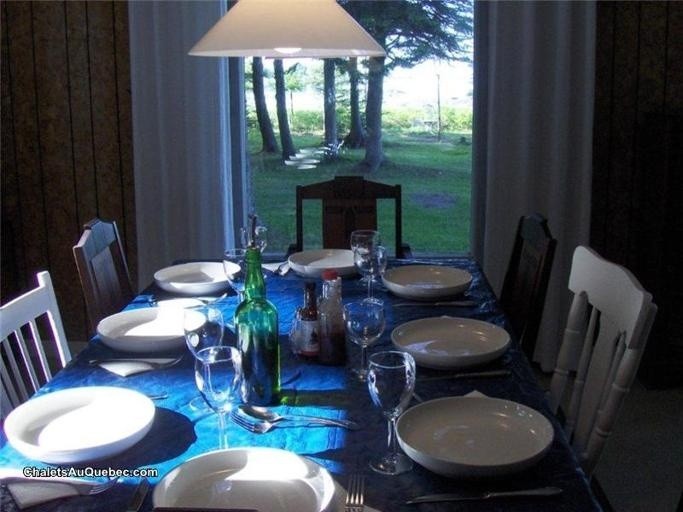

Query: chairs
left=72, top=216, right=137, bottom=338
left=498, top=212, right=557, bottom=367
left=1, top=269, right=74, bottom=422
left=295, top=177, right=403, bottom=259
left=547, top=244, right=658, bottom=474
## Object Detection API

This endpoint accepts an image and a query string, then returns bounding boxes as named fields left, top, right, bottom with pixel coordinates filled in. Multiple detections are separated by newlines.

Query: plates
left=152, top=446, right=336, bottom=511
left=153, top=260, right=241, bottom=295
left=3, top=386, right=155, bottom=467
left=381, top=265, right=473, bottom=299
left=396, top=396, right=554, bottom=477
left=288, top=248, right=356, bottom=278
left=97, top=308, right=206, bottom=354
left=391, top=315, right=512, bottom=370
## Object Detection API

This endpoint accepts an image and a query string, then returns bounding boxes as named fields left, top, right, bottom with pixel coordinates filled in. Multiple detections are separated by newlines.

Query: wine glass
left=183, top=308, right=223, bottom=412
left=223, top=248, right=246, bottom=327
left=367, top=350, right=416, bottom=475
left=195, top=346, right=243, bottom=450
left=240, top=225, right=268, bottom=280
left=343, top=229, right=385, bottom=381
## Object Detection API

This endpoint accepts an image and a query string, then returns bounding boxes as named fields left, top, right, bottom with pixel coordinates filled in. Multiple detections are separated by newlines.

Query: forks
left=231, top=410, right=327, bottom=433
left=344, top=472, right=367, bottom=511
left=0, top=476, right=119, bottom=495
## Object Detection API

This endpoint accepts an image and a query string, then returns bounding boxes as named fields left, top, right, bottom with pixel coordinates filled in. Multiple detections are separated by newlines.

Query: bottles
left=236, top=249, right=281, bottom=407
left=318, top=269, right=346, bottom=366
left=295, top=282, right=318, bottom=357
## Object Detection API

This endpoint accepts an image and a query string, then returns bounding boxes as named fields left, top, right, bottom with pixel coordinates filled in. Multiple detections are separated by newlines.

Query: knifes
left=405, top=486, right=564, bottom=504
left=417, top=367, right=511, bottom=382
left=392, top=300, right=479, bottom=307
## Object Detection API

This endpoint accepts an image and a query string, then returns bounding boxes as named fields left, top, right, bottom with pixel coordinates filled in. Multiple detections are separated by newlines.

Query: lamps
left=187, top=0, right=388, bottom=59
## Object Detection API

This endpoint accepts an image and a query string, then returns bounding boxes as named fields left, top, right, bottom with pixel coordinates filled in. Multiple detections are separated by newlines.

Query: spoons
left=240, top=405, right=360, bottom=432
